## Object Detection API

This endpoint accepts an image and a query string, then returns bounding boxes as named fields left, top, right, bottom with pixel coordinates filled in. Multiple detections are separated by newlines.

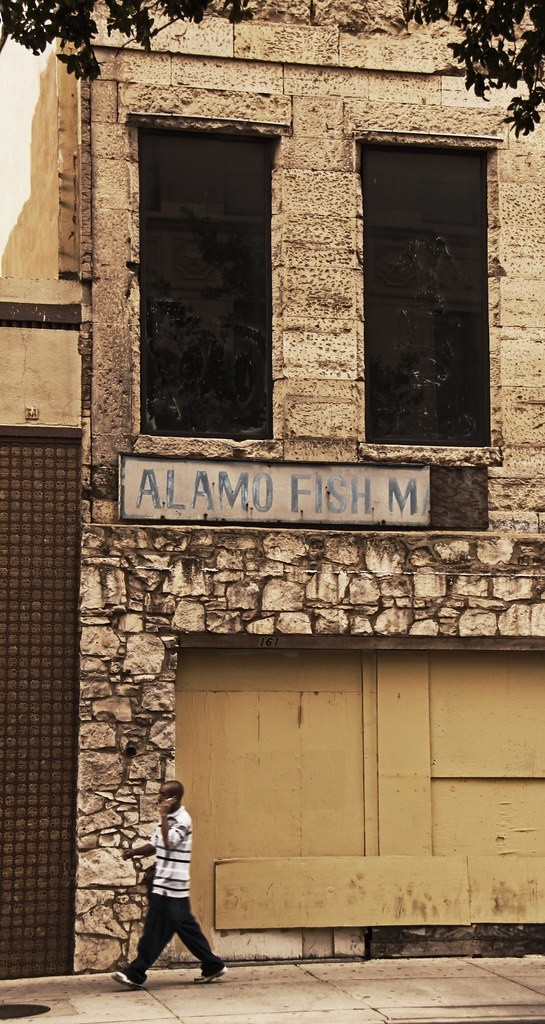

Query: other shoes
left=112, top=971, right=143, bottom=991
left=193, top=966, right=227, bottom=983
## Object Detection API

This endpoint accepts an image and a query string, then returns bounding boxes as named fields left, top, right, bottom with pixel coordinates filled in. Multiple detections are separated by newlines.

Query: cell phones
left=163, top=798, right=177, bottom=804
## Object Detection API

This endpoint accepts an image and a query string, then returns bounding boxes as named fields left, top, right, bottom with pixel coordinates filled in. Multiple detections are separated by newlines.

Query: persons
left=111, top=781, right=227, bottom=989
